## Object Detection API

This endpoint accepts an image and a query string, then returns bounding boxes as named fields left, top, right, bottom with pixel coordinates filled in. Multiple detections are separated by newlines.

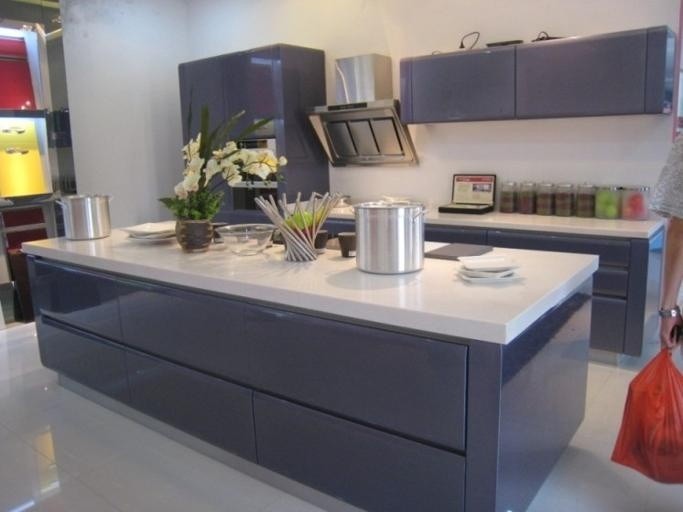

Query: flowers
left=153, top=77, right=288, bottom=223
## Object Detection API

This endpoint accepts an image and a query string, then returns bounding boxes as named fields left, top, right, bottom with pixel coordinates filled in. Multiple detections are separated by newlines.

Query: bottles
left=497, top=179, right=651, bottom=221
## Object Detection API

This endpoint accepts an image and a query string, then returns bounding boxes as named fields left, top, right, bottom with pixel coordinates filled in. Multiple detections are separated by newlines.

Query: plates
left=454, top=252, right=520, bottom=283
left=119, top=223, right=176, bottom=245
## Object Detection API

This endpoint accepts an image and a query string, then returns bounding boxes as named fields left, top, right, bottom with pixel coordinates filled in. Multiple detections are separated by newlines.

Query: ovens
left=227, top=135, right=279, bottom=211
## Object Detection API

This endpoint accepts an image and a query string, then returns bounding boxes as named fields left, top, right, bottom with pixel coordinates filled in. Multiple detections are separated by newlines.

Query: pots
left=346, top=199, right=432, bottom=275
left=53, top=193, right=111, bottom=239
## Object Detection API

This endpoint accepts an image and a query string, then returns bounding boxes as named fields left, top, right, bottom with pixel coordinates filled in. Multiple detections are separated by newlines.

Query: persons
left=649, top=129, right=683, bottom=355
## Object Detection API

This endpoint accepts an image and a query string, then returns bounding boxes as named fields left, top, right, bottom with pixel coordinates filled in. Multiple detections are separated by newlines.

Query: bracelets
left=658, top=307, right=681, bottom=318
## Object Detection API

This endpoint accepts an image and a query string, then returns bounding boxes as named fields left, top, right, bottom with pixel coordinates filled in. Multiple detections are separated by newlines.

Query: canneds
left=500, top=182, right=649, bottom=222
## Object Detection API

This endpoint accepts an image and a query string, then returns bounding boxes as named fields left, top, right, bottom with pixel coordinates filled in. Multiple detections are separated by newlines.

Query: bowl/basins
left=214, top=222, right=276, bottom=255
left=331, top=195, right=351, bottom=208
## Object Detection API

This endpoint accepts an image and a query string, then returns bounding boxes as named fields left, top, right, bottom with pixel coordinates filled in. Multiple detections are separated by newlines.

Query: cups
left=311, top=229, right=329, bottom=254
left=272, top=231, right=283, bottom=243
left=211, top=222, right=226, bottom=244
left=339, top=233, right=357, bottom=258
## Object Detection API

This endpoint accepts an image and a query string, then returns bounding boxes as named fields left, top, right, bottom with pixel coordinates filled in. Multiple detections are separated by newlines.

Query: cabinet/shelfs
left=17, top=218, right=668, bottom=512
left=392, top=23, right=676, bottom=138
left=224, top=41, right=329, bottom=205
left=178, top=56, right=233, bottom=225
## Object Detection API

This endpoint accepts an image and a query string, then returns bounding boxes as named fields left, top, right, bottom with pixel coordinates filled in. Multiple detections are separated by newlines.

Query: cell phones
left=674, top=326, right=679, bottom=344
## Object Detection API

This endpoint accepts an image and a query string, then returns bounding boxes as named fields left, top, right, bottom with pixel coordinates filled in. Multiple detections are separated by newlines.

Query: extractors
left=304, top=53, right=421, bottom=170
